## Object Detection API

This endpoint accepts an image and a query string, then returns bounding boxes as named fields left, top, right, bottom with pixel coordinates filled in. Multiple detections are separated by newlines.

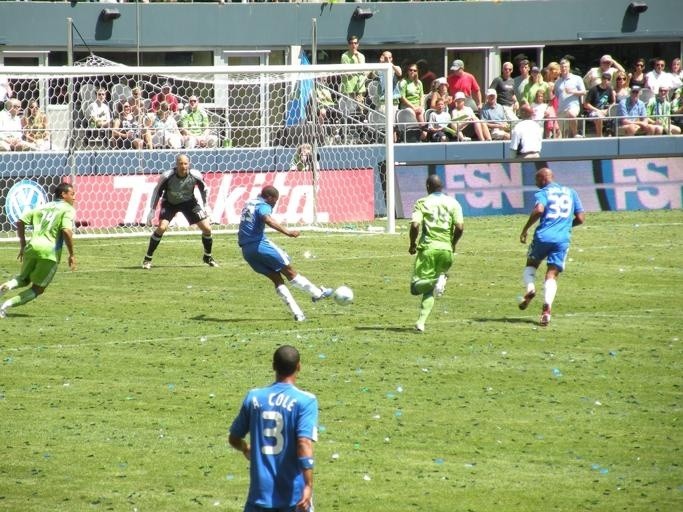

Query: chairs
left=317, top=74, right=682, bottom=138
left=21, top=83, right=218, bottom=151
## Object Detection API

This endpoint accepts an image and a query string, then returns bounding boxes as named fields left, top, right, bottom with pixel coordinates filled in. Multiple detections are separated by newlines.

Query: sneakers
left=294, top=315, right=306, bottom=321
left=312, top=287, right=333, bottom=301
left=433, top=274, right=448, bottom=297
left=542, top=314, right=551, bottom=324
left=519, top=289, right=536, bottom=310
left=204, top=256, right=218, bottom=267
left=142, top=260, right=151, bottom=269
left=416, top=321, right=425, bottom=330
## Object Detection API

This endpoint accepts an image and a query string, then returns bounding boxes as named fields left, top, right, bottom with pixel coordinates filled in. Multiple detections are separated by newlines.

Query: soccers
left=333, top=286, right=354, bottom=306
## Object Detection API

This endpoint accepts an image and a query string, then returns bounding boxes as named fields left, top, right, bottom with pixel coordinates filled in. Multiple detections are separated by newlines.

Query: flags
left=283, top=52, right=313, bottom=127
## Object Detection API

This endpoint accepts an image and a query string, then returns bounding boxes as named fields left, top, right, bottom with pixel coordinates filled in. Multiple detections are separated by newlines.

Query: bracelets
left=298, top=455, right=313, bottom=470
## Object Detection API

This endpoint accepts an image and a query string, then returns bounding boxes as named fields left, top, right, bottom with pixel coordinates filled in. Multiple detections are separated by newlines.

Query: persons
left=0, top=182, right=76, bottom=318
left=87, top=86, right=218, bottom=150
left=289, top=143, right=313, bottom=172
left=509, top=102, right=543, bottom=160
left=19, top=100, right=53, bottom=152
left=517, top=166, right=586, bottom=326
left=0, top=78, right=12, bottom=112
left=0, top=98, right=28, bottom=152
left=140, top=153, right=218, bottom=269
left=228, top=345, right=320, bottom=512
left=403, top=175, right=463, bottom=331
left=239, top=185, right=333, bottom=321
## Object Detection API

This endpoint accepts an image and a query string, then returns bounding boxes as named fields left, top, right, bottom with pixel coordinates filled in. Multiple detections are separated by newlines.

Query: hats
left=435, top=55, right=641, bottom=113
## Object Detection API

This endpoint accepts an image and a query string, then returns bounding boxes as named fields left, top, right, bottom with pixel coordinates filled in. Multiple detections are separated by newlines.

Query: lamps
left=353, top=7, right=375, bottom=20
left=630, top=1, right=651, bottom=13
left=101, top=6, right=122, bottom=20
